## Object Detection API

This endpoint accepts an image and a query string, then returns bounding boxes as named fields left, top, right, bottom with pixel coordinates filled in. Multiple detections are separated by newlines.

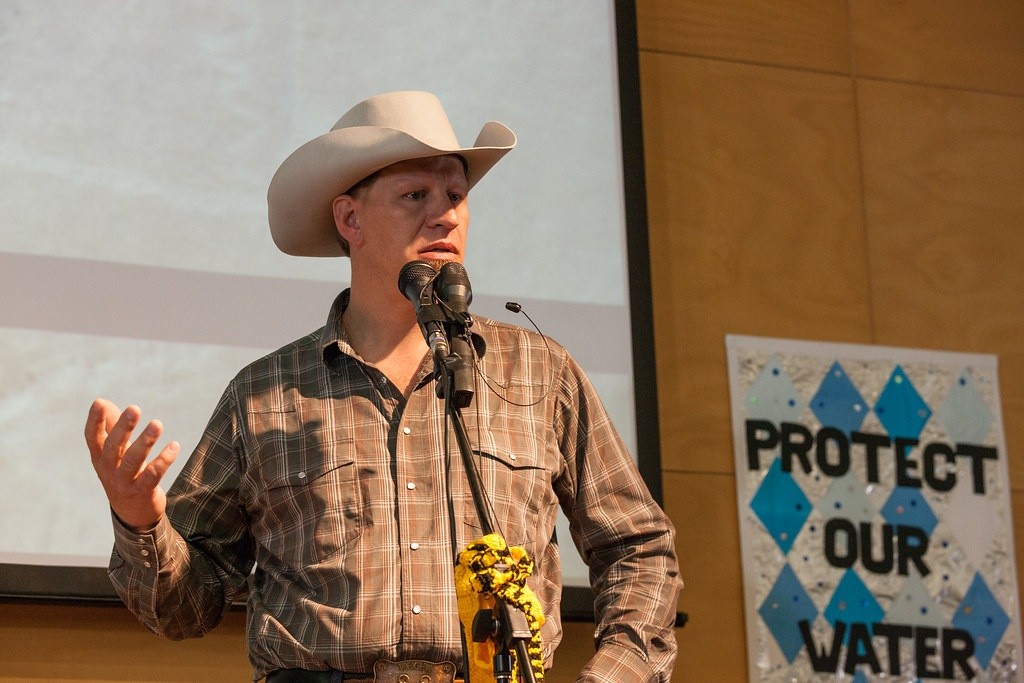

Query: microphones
left=504, top=302, right=521, bottom=313
left=397, top=260, right=475, bottom=411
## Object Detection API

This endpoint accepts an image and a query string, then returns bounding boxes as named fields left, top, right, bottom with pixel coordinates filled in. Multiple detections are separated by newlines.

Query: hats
left=268, top=89, right=517, bottom=258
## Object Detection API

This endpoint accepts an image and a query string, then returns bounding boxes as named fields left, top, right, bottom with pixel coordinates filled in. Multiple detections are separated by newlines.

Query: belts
left=259, top=656, right=468, bottom=683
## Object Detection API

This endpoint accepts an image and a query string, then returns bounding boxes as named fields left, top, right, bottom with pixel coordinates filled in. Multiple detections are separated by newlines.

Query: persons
left=85, top=92, right=684, bottom=683
